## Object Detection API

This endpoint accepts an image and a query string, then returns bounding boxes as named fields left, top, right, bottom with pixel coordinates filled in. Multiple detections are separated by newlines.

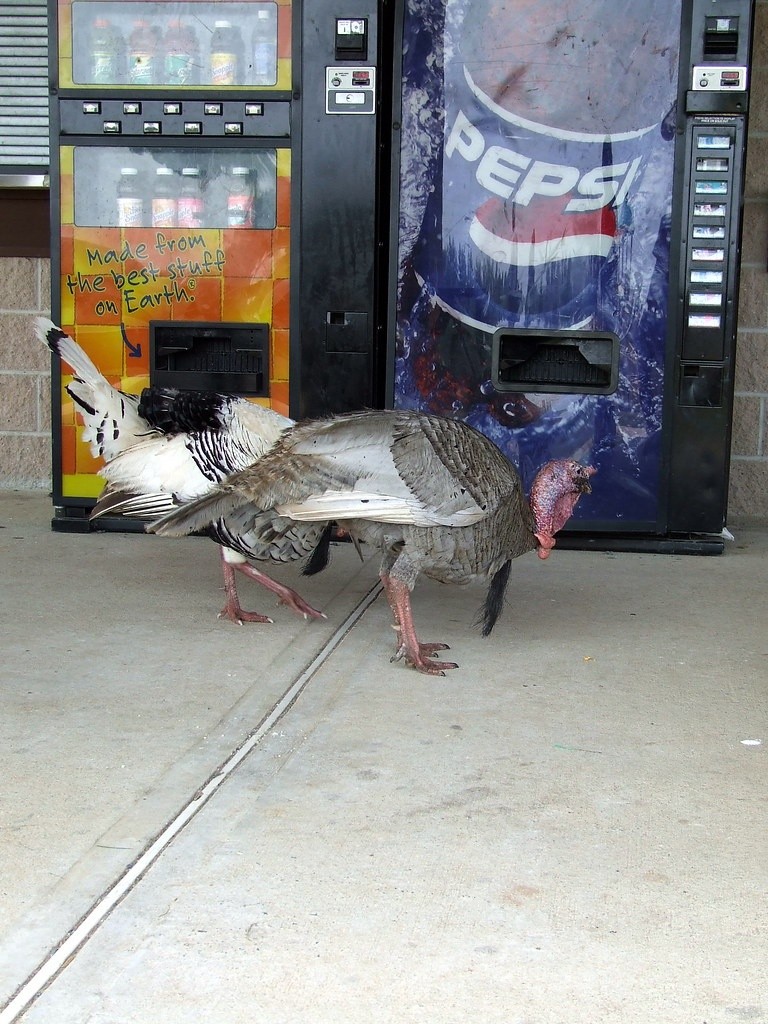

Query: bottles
left=116, top=168, right=142, bottom=227
left=401, top=0, right=690, bottom=421
left=162, top=19, right=193, bottom=84
left=151, top=168, right=179, bottom=228
left=227, top=168, right=255, bottom=228
left=127, top=20, right=157, bottom=84
left=251, top=10, right=276, bottom=84
left=209, top=21, right=237, bottom=85
left=179, top=168, right=205, bottom=228
left=89, top=18, right=116, bottom=83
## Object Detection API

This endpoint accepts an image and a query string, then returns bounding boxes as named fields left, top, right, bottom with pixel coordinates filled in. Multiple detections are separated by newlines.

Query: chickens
left=143, top=410, right=594, bottom=677
left=33, top=316, right=364, bottom=625
